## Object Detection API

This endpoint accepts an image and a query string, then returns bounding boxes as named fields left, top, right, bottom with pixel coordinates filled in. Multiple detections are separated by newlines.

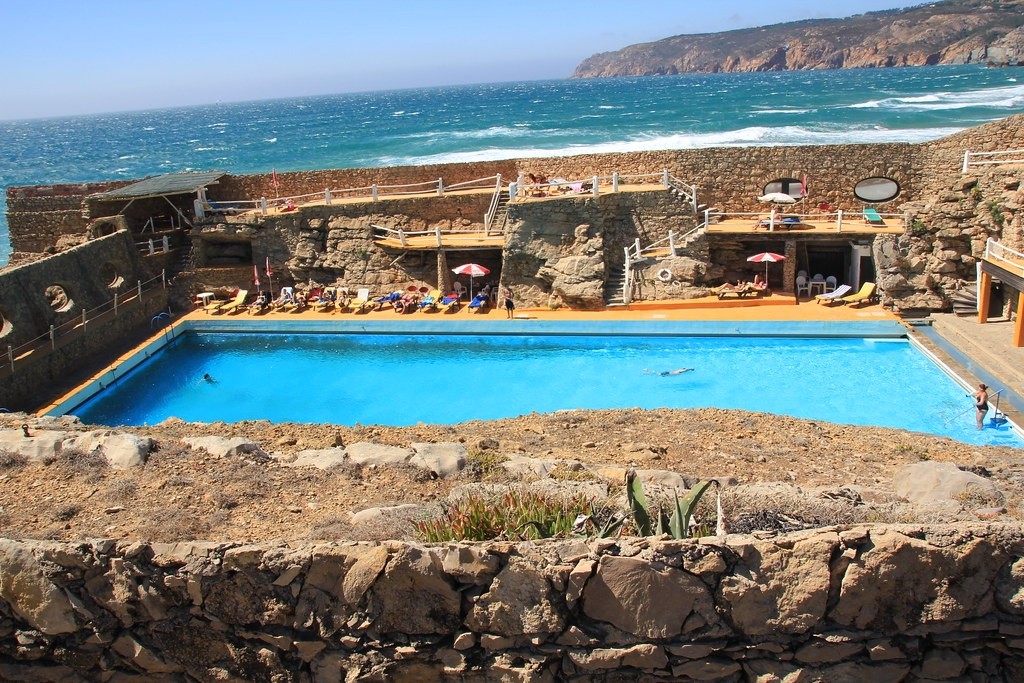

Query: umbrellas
left=747, top=252, right=786, bottom=285
left=800, top=174, right=808, bottom=225
left=758, top=192, right=797, bottom=208
left=254, top=265, right=261, bottom=292
left=266, top=257, right=275, bottom=302
left=273, top=168, right=280, bottom=204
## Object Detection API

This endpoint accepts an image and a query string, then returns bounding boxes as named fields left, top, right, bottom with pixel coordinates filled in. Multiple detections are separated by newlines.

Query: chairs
left=760, top=214, right=803, bottom=230
left=204, top=286, right=490, bottom=316
left=453, top=282, right=468, bottom=300
left=864, top=208, right=882, bottom=227
left=549, top=176, right=594, bottom=193
left=796, top=272, right=875, bottom=308
left=712, top=282, right=765, bottom=299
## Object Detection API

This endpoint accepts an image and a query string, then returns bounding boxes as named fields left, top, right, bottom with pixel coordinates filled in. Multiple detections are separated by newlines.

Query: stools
left=194, top=300, right=203, bottom=307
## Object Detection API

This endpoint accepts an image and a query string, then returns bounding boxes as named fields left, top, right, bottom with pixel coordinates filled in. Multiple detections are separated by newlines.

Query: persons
left=502, top=282, right=514, bottom=319
left=295, top=289, right=306, bottom=305
left=319, top=289, right=332, bottom=302
left=752, top=208, right=782, bottom=230
left=973, top=384, right=989, bottom=428
left=754, top=271, right=764, bottom=287
left=204, top=374, right=210, bottom=379
left=338, top=291, right=346, bottom=307
left=389, top=291, right=415, bottom=314
left=252, top=290, right=265, bottom=306
left=718, top=280, right=746, bottom=291
left=271, top=289, right=288, bottom=306
left=452, top=263, right=490, bottom=302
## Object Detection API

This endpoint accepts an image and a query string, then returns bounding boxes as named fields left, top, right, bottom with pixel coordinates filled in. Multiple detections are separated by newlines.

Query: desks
left=196, top=292, right=216, bottom=306
left=809, top=279, right=827, bottom=298
left=528, top=186, right=548, bottom=197
